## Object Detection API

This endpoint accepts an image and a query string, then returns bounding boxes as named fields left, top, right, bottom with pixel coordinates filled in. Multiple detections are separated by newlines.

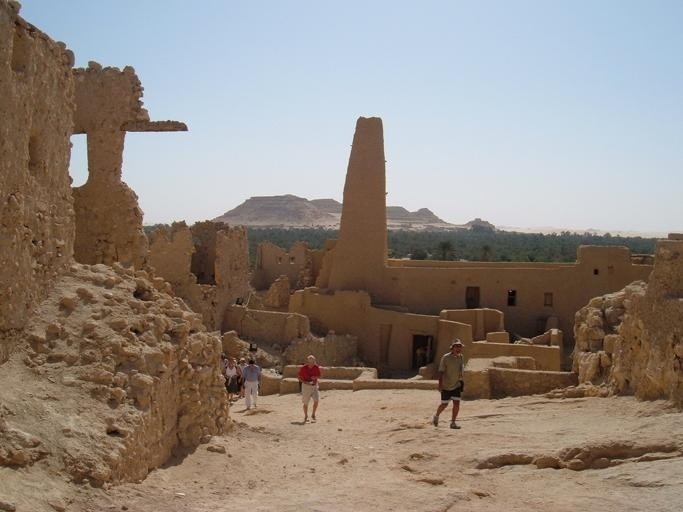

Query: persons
left=296, top=353, right=321, bottom=423
left=222, top=358, right=228, bottom=366
left=242, top=359, right=261, bottom=409
left=219, top=357, right=242, bottom=403
left=237, top=358, right=249, bottom=399
left=432, top=338, right=465, bottom=429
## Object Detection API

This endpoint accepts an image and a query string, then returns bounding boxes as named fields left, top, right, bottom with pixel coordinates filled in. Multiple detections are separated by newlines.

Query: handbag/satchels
left=459, top=379, right=465, bottom=392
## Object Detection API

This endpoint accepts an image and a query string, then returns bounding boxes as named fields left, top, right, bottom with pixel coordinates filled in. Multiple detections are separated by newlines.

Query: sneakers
left=449, top=423, right=461, bottom=429
left=432, top=414, right=439, bottom=427
left=304, top=415, right=316, bottom=422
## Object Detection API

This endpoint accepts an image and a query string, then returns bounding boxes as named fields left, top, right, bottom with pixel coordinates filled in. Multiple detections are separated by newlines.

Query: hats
left=449, top=338, right=464, bottom=349
left=247, top=357, right=255, bottom=363
